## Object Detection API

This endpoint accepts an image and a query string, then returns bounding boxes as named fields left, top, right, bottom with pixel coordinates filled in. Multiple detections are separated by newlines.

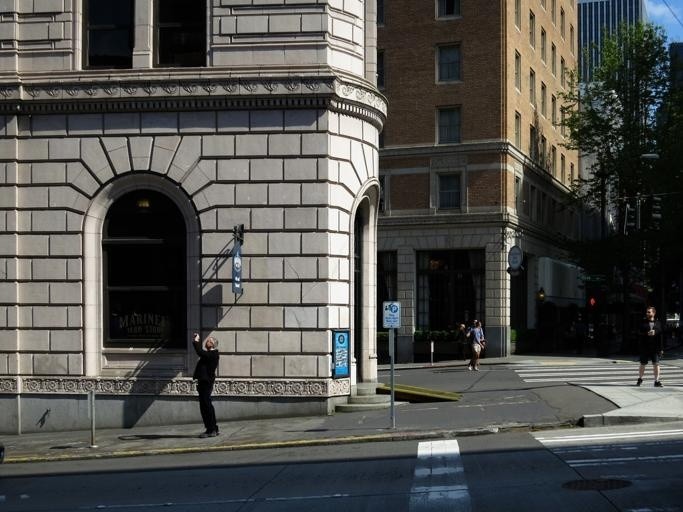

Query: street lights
left=601, top=152, right=659, bottom=272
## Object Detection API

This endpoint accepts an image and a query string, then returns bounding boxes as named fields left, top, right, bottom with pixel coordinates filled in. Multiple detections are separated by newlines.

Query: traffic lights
left=584, top=279, right=601, bottom=312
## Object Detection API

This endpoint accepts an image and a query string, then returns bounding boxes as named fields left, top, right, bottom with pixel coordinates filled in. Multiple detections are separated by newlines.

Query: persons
left=637, top=306, right=663, bottom=387
left=191, top=332, right=220, bottom=439
left=459, top=320, right=486, bottom=371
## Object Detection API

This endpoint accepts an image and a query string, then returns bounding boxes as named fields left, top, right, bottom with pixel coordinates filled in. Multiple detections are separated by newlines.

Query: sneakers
left=635, top=378, right=664, bottom=388
left=468, top=365, right=479, bottom=371
left=199, top=429, right=220, bottom=438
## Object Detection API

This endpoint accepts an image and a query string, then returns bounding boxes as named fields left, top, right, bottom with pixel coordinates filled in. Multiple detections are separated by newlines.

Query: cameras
left=193, top=332, right=197, bottom=337
